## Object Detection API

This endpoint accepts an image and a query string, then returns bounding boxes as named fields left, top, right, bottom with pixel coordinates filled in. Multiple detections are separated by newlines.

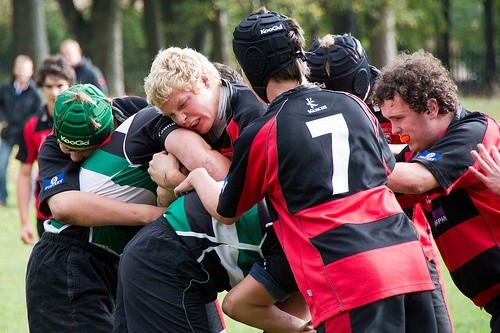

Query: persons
left=0, top=7, right=500, bottom=333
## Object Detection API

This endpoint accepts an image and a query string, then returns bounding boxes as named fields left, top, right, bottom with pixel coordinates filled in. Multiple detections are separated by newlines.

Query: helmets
left=54, top=84, right=114, bottom=150
left=233, top=10, right=297, bottom=92
left=304, top=33, right=371, bottom=101
